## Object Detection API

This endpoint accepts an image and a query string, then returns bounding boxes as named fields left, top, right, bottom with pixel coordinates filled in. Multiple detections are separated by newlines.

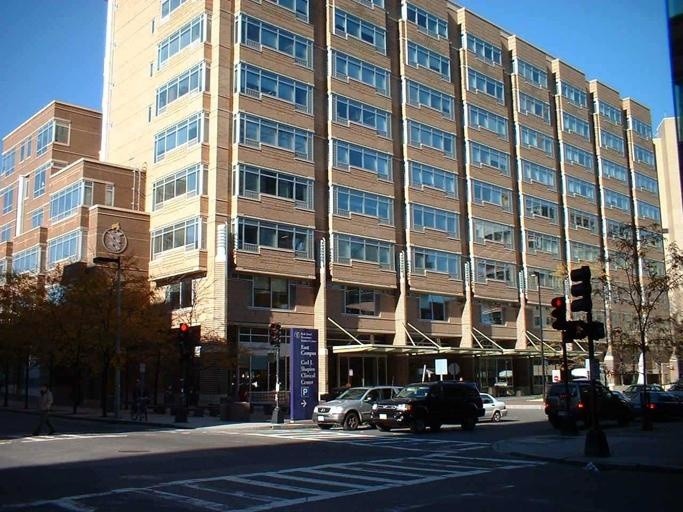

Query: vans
left=368, top=381, right=486, bottom=437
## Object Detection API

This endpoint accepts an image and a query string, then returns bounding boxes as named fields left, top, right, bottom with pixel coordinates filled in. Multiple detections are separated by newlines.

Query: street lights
left=527, top=269, right=545, bottom=404
left=91, top=252, right=123, bottom=419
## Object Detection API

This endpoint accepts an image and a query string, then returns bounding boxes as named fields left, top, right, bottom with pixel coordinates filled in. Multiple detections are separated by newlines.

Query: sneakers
left=32, top=431, right=56, bottom=435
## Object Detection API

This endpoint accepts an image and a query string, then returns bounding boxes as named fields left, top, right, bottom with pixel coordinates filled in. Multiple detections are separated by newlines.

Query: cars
left=474, top=392, right=508, bottom=423
left=617, top=381, right=682, bottom=424
left=308, top=383, right=403, bottom=432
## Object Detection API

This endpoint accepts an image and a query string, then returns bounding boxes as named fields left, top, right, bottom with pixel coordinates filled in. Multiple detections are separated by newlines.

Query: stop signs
left=553, top=375, right=558, bottom=381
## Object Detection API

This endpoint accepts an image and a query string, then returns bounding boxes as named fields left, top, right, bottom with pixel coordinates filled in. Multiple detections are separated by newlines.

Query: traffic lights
left=268, top=322, right=280, bottom=347
left=567, top=320, right=585, bottom=338
left=178, top=323, right=187, bottom=347
left=569, top=264, right=593, bottom=312
left=549, top=295, right=566, bottom=331
left=562, top=331, right=573, bottom=343
left=586, top=319, right=604, bottom=342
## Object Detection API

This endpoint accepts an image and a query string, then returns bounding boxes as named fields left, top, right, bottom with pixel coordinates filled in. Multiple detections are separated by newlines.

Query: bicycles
left=128, top=394, right=151, bottom=421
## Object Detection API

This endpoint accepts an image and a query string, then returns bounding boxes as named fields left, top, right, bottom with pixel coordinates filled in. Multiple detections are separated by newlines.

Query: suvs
left=542, top=379, right=632, bottom=432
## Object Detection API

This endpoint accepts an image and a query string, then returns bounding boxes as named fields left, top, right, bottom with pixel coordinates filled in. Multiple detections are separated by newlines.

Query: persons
left=30, top=379, right=55, bottom=435
left=173, top=377, right=184, bottom=408
left=132, top=375, right=149, bottom=421
left=163, top=383, right=172, bottom=417
